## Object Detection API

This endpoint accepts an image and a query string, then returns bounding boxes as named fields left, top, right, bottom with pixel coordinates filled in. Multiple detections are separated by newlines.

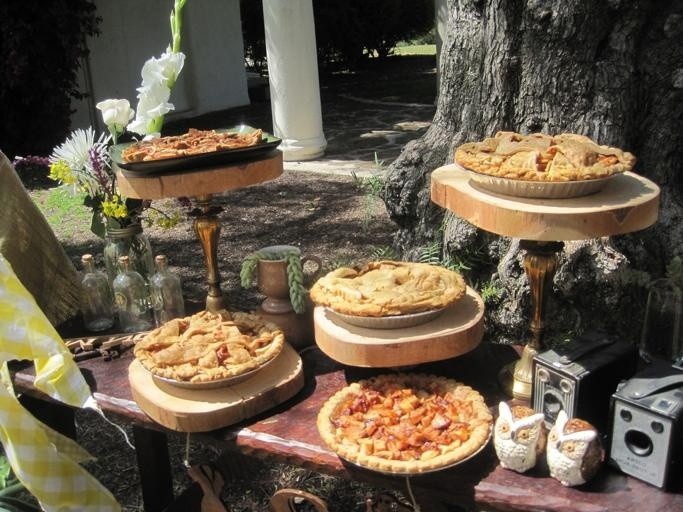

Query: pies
left=311, top=261, right=465, bottom=319
left=120, top=125, right=262, bottom=162
left=134, top=310, right=285, bottom=382
left=455, top=130, right=636, bottom=183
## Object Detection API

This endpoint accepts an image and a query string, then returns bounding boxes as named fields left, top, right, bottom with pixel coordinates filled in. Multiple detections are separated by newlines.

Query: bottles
left=149, top=254, right=186, bottom=327
left=112, top=256, right=152, bottom=335
left=77, top=254, right=114, bottom=332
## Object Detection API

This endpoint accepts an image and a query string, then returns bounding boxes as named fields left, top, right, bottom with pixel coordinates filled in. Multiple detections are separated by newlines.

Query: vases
left=105, top=225, right=154, bottom=291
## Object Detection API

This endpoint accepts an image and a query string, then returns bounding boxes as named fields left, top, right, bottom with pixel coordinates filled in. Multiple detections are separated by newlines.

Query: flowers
left=46, top=9, right=187, bottom=270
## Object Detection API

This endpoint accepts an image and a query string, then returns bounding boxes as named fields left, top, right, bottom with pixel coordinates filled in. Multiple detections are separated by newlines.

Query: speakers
left=530, top=330, right=634, bottom=431
left=610, top=362, right=683, bottom=490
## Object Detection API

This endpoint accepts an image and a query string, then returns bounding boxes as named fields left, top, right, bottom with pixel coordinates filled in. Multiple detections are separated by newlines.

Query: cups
left=257, top=244, right=322, bottom=314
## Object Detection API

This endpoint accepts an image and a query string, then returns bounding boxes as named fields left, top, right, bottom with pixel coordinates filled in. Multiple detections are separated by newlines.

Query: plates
left=324, top=302, right=466, bottom=329
left=133, top=335, right=285, bottom=390
left=454, top=154, right=637, bottom=198
left=336, top=420, right=491, bottom=477
left=109, top=123, right=282, bottom=173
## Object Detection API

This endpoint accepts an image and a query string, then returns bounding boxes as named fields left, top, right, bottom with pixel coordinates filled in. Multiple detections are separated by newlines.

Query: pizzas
left=315, top=373, right=493, bottom=474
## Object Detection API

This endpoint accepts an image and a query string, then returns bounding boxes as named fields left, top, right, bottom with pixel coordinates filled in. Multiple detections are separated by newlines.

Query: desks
left=5, top=314, right=676, bottom=510
left=430, top=160, right=661, bottom=356
left=113, top=152, right=283, bottom=311
